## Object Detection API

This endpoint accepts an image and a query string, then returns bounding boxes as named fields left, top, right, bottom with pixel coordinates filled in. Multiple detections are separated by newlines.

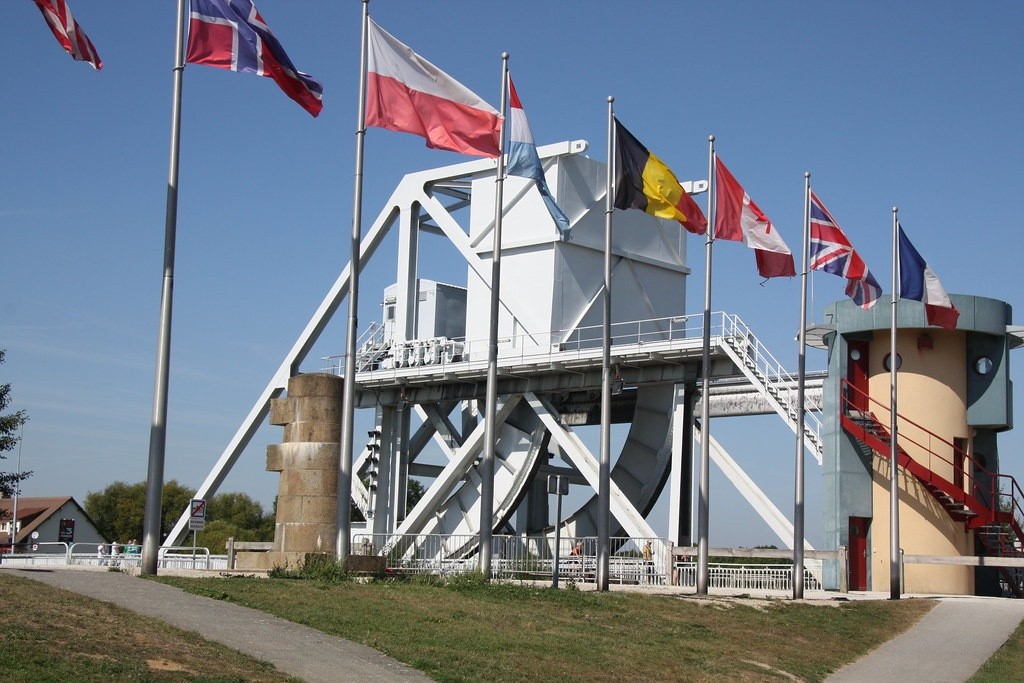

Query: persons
left=573, top=542, right=584, bottom=556
left=111, top=542, right=123, bottom=566
left=125, top=540, right=131, bottom=557
left=97, top=543, right=106, bottom=566
left=128, top=539, right=138, bottom=554
left=643, top=541, right=652, bottom=559
left=360, top=538, right=375, bottom=556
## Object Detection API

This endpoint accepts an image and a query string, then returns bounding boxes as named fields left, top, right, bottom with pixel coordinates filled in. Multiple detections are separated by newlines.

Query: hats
left=648, top=540, right=652, bottom=543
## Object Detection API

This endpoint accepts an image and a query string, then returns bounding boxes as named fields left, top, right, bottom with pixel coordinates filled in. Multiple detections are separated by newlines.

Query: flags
left=897, top=222, right=960, bottom=332
left=182, top=0, right=324, bottom=117
left=713, top=153, right=797, bottom=277
left=614, top=116, right=708, bottom=235
left=366, top=16, right=502, bottom=159
left=35, top=0, right=103, bottom=71
left=505, top=71, right=573, bottom=242
left=809, top=191, right=882, bottom=310
